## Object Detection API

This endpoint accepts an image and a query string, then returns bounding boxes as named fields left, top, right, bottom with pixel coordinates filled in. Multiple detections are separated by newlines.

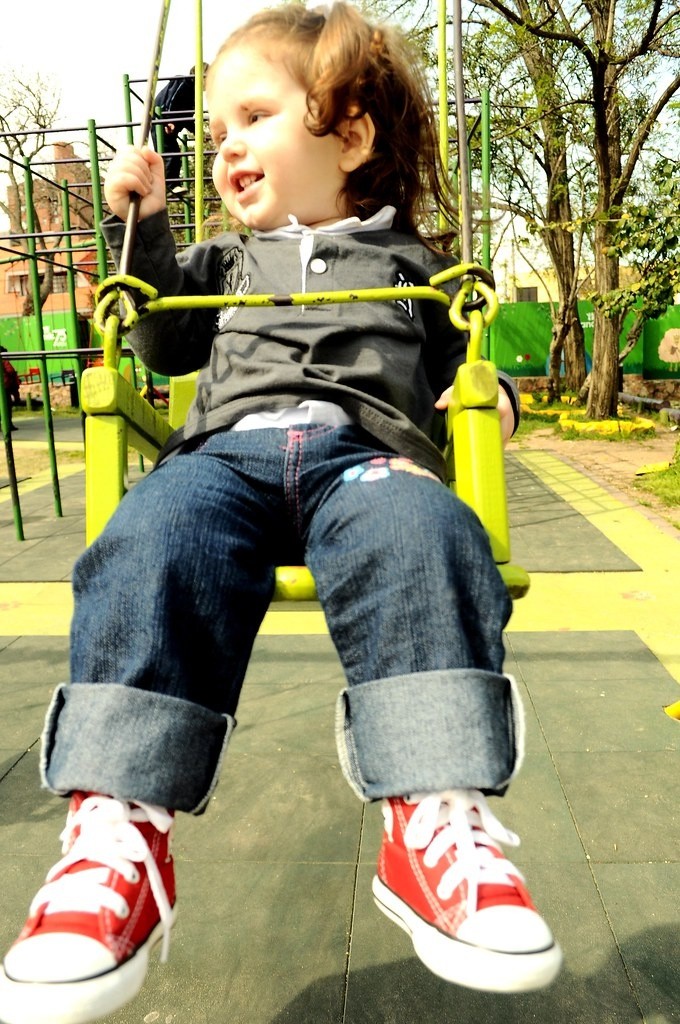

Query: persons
left=1, top=2, right=579, bottom=1023
left=0, top=345, right=22, bottom=433
left=145, top=61, right=214, bottom=195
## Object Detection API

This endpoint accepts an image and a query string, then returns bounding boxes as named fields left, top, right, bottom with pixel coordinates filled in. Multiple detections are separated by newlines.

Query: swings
left=80, top=1, right=533, bottom=598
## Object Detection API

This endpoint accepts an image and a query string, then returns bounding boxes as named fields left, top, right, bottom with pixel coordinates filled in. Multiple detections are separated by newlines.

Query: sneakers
left=369, top=793, right=562, bottom=994
left=0, top=793, right=179, bottom=1023
